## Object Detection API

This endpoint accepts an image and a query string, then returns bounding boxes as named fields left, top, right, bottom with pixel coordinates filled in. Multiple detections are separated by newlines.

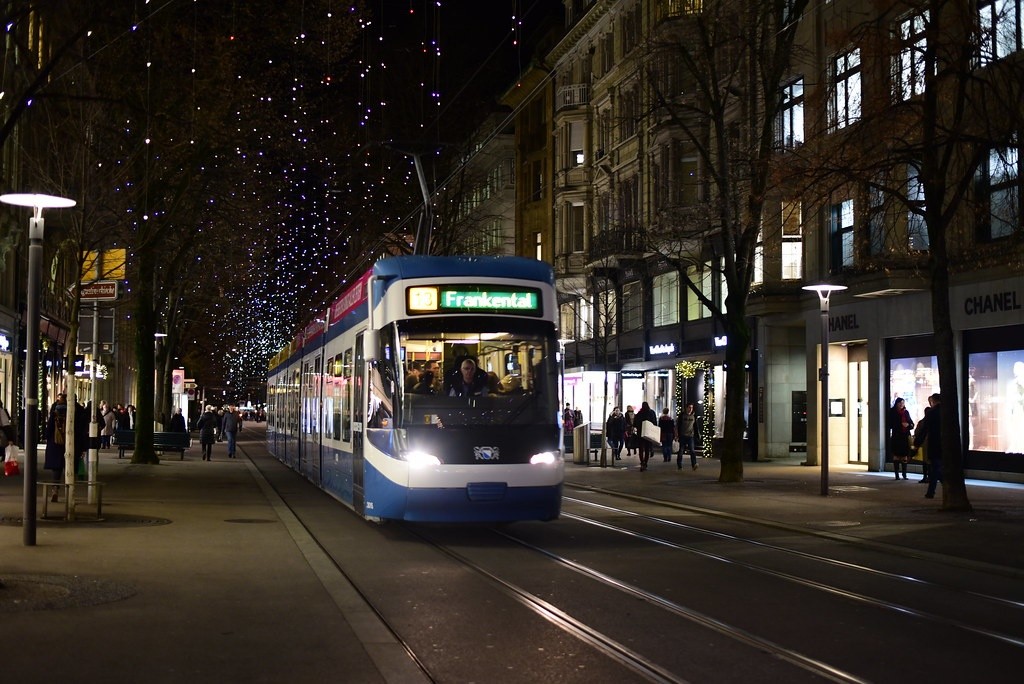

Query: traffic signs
left=65, top=280, right=118, bottom=301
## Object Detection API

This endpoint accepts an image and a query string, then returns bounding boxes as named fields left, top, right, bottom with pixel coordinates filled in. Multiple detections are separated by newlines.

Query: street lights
left=556, top=339, right=575, bottom=421
left=0, top=192, right=77, bottom=545
left=802, top=283, right=848, bottom=496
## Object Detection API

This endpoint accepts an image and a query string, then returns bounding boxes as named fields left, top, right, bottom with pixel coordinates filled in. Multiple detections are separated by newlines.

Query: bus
left=266, top=253, right=565, bottom=522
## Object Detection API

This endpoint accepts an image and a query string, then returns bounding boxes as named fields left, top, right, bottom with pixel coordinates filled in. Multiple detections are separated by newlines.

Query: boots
left=51, top=486, right=58, bottom=502
left=902, top=462, right=909, bottom=480
left=894, top=460, right=901, bottom=480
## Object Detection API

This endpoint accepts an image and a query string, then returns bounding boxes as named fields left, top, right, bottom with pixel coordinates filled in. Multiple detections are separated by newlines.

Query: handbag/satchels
left=641, top=420, right=661, bottom=446
left=218, top=434, right=223, bottom=442
left=4, top=445, right=21, bottom=478
left=671, top=439, right=680, bottom=453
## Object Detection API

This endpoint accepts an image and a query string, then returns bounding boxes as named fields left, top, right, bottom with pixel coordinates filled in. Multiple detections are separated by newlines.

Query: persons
left=45, top=393, right=105, bottom=502
left=211, top=407, right=227, bottom=444
left=565, top=403, right=583, bottom=433
left=197, top=404, right=216, bottom=461
left=606, top=407, right=627, bottom=460
left=913, top=394, right=942, bottom=499
left=405, top=360, right=442, bottom=393
left=171, top=408, right=185, bottom=432
left=0, top=399, right=14, bottom=447
left=658, top=408, right=675, bottom=462
left=452, top=359, right=524, bottom=397
left=624, top=405, right=637, bottom=458
left=221, top=404, right=243, bottom=458
left=100, top=400, right=136, bottom=449
left=675, top=403, right=702, bottom=470
left=886, top=397, right=916, bottom=479
left=633, top=402, right=657, bottom=471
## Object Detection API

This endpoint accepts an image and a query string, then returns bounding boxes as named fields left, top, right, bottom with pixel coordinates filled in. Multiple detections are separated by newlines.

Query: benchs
left=564, top=434, right=602, bottom=461
left=113, top=429, right=190, bottom=460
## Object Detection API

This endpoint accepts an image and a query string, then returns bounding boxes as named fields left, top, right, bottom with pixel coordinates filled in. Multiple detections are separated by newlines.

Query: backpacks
left=54, top=413, right=65, bottom=445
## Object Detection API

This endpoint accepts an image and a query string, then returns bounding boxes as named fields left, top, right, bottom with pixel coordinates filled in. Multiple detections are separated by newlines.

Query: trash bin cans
left=573, top=421, right=590, bottom=465
left=154, top=420, right=163, bottom=455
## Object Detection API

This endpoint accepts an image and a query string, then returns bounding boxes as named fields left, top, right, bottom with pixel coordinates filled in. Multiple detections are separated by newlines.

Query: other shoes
left=107, top=444, right=110, bottom=449
left=627, top=453, right=631, bottom=456
left=643, top=464, right=647, bottom=470
left=678, top=468, right=683, bottom=472
left=919, top=478, right=929, bottom=482
left=664, top=459, right=667, bottom=462
left=634, top=449, right=636, bottom=454
left=925, top=489, right=934, bottom=499
left=650, top=451, right=654, bottom=458
left=640, top=467, right=643, bottom=472
left=233, top=456, right=236, bottom=458
left=228, top=454, right=232, bottom=458
left=202, top=453, right=206, bottom=460
left=693, top=463, right=699, bottom=471
left=102, top=445, right=106, bottom=449
left=207, top=458, right=211, bottom=461
left=668, top=455, right=671, bottom=462
